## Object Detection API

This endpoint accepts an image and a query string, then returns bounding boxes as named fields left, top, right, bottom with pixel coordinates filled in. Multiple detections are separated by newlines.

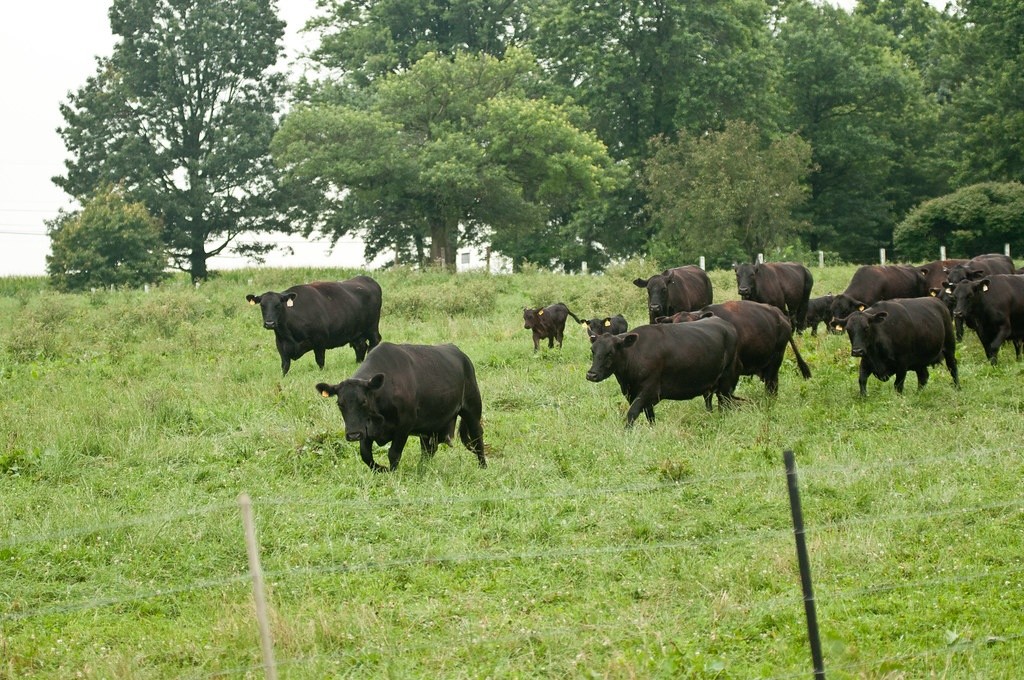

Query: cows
left=523, top=302, right=581, bottom=353
left=315, top=342, right=489, bottom=473
left=246, top=276, right=382, bottom=376
left=580, top=252, right=1024, bottom=430
